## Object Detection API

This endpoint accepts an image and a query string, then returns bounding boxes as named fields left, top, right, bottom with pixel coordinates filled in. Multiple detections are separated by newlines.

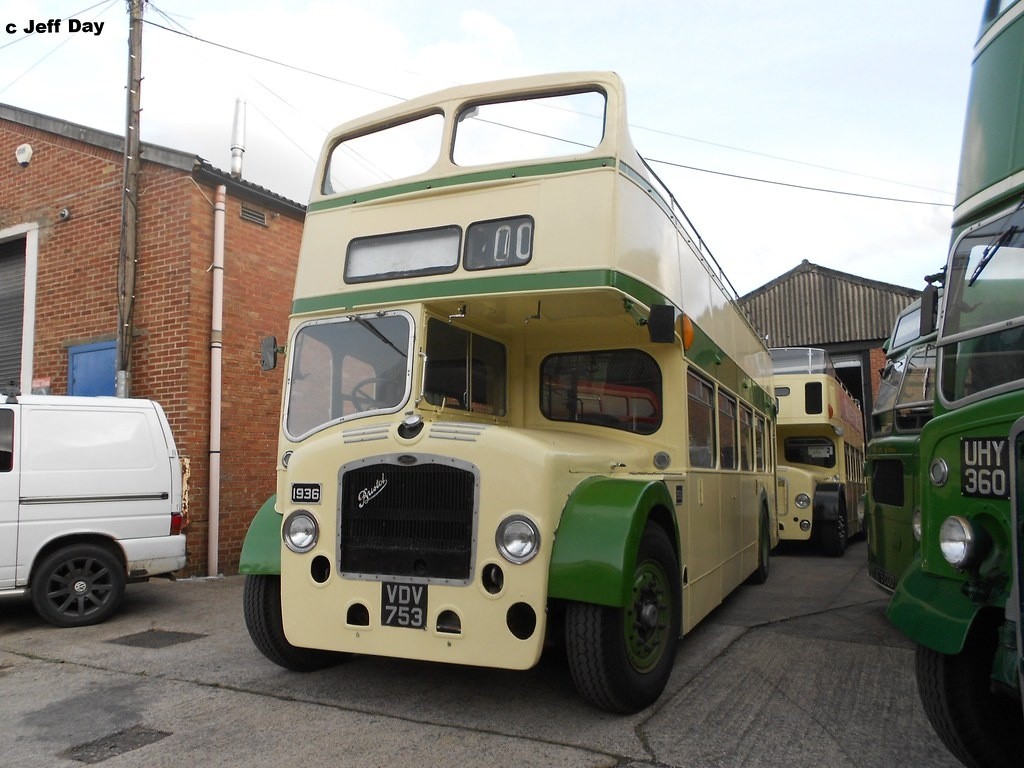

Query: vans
left=0, top=392, right=188, bottom=627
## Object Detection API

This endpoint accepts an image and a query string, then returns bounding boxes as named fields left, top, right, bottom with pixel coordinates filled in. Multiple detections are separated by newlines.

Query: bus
left=766, top=346, right=868, bottom=558
left=242, top=73, right=782, bottom=717
left=863, top=292, right=943, bottom=595
left=888, top=0, right=1024, bottom=767
